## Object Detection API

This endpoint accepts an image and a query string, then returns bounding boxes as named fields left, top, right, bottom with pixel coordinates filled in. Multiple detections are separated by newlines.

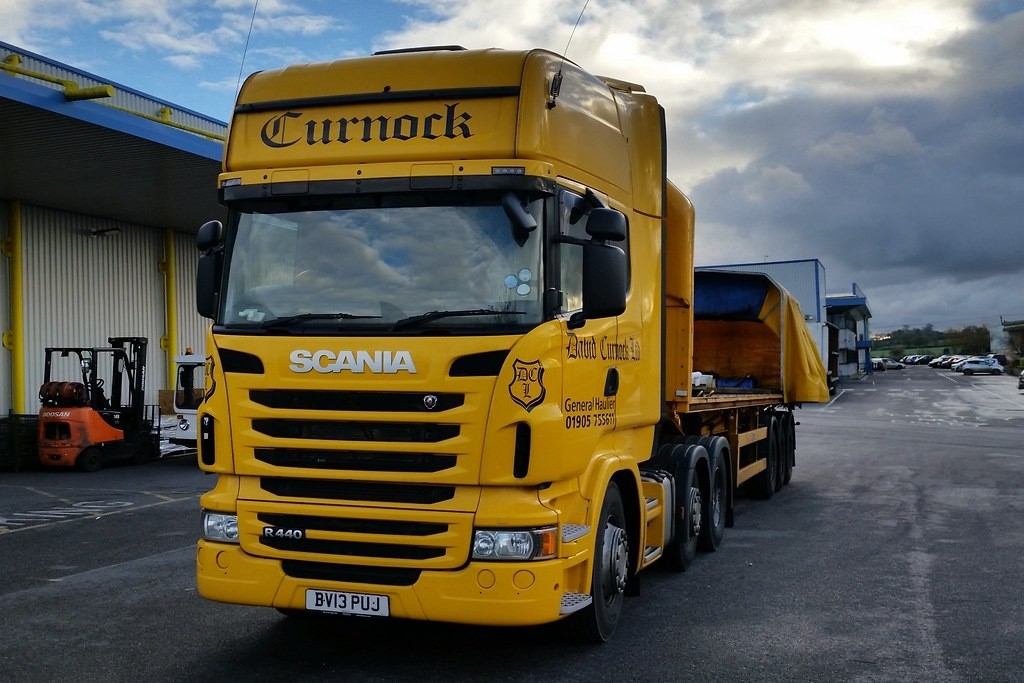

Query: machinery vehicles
left=34, top=336, right=162, bottom=470
left=166, top=347, right=210, bottom=449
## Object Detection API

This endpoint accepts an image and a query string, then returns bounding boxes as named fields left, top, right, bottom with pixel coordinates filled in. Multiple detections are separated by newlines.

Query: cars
left=960, top=360, right=1005, bottom=375
left=871, top=358, right=906, bottom=370
left=900, top=355, right=935, bottom=365
left=930, top=356, right=987, bottom=370
left=1018, top=369, right=1024, bottom=389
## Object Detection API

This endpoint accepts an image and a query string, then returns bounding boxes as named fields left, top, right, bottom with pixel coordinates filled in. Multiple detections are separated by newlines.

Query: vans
left=987, top=354, right=1006, bottom=366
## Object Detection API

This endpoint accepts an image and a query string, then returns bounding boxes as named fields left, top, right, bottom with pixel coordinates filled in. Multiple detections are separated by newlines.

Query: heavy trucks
left=195, top=44, right=831, bottom=645
